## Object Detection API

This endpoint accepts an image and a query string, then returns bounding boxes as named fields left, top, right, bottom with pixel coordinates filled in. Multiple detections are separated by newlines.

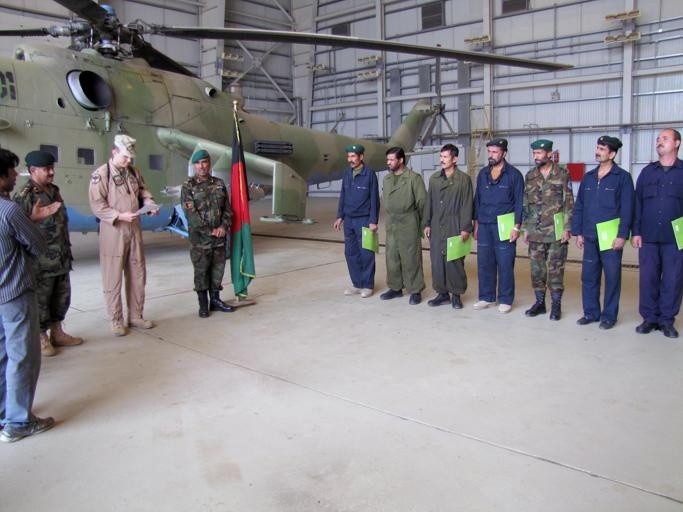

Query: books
left=496, top=211, right=525, bottom=243
left=553, top=211, right=565, bottom=242
left=595, top=218, right=631, bottom=253
left=671, top=216, right=683, bottom=251
left=361, top=225, right=379, bottom=255
left=446, top=233, right=472, bottom=262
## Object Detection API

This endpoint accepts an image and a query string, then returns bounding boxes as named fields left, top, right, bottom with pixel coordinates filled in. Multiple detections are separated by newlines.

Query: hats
left=114, top=134, right=137, bottom=158
left=486, top=138, right=507, bottom=147
left=192, top=150, right=209, bottom=163
left=25, top=150, right=55, bottom=167
left=531, top=140, right=553, bottom=149
left=345, top=144, right=365, bottom=154
left=597, top=136, right=622, bottom=147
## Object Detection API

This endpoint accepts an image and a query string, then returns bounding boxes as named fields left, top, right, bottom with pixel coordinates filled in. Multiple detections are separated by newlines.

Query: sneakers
left=577, top=318, right=678, bottom=338
left=128, top=319, right=153, bottom=329
left=345, top=287, right=511, bottom=314
left=0, top=417, right=56, bottom=443
left=112, top=327, right=126, bottom=336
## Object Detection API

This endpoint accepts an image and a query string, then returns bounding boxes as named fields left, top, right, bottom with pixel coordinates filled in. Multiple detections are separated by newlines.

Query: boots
left=50, top=322, right=82, bottom=345
left=550, top=292, right=562, bottom=321
left=40, top=331, right=56, bottom=356
left=526, top=291, right=546, bottom=316
left=197, top=289, right=209, bottom=318
left=209, top=290, right=233, bottom=312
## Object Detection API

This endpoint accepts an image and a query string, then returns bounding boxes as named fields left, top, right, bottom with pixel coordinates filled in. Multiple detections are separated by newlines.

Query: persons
left=379, top=147, right=428, bottom=305
left=331, top=145, right=380, bottom=300
left=422, top=144, right=474, bottom=309
left=469, top=137, right=524, bottom=314
left=0, top=147, right=63, bottom=445
left=87, top=134, right=162, bottom=338
left=629, top=128, right=683, bottom=338
left=520, top=139, right=575, bottom=320
left=9, top=150, right=83, bottom=357
left=177, top=149, right=235, bottom=319
left=570, top=135, right=635, bottom=330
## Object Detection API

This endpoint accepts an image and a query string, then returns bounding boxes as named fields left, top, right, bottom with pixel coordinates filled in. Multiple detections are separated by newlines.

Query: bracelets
left=513, top=227, right=521, bottom=233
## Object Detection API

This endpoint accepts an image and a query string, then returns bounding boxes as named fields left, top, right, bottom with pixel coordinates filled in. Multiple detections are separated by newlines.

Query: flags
left=230, top=110, right=254, bottom=303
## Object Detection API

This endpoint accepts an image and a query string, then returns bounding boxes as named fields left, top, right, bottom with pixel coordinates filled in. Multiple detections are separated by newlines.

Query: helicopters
left=0, top=2, right=577, bottom=237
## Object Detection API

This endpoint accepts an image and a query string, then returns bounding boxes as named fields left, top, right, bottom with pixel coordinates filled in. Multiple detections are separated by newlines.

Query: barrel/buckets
left=566, top=161, right=586, bottom=181
